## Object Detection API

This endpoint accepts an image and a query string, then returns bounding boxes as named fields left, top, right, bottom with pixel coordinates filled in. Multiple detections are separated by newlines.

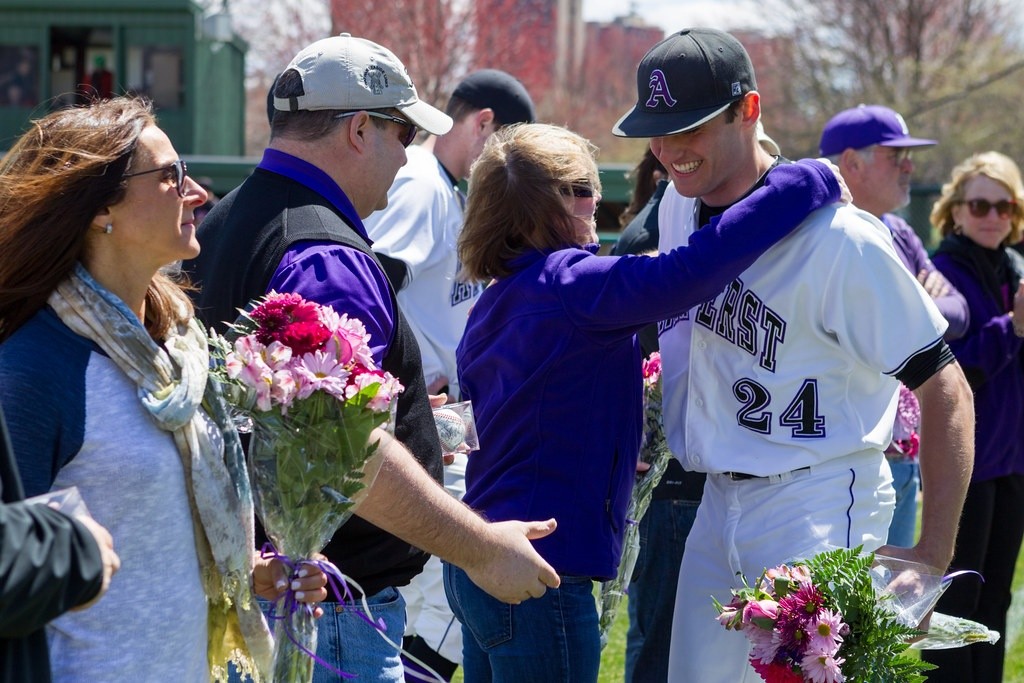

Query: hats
left=817, top=103, right=936, bottom=158
left=274, top=31, right=454, bottom=140
left=611, top=29, right=757, bottom=139
left=451, top=69, right=536, bottom=124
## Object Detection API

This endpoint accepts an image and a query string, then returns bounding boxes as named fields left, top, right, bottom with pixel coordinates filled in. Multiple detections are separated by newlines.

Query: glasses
left=562, top=179, right=593, bottom=199
left=958, top=198, right=1017, bottom=224
left=122, top=159, right=186, bottom=198
left=894, top=149, right=914, bottom=162
left=334, top=109, right=416, bottom=150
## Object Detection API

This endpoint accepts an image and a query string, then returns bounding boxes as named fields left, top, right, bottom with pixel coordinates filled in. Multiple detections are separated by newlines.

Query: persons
left=0, top=25, right=1023, bottom=683
left=180, top=33, right=563, bottom=683
left=362, top=66, right=536, bottom=683
left=442, top=124, right=853, bottom=683
left=820, top=105, right=971, bottom=548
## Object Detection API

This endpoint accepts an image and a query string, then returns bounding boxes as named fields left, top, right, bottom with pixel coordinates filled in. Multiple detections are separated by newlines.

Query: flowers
left=596, top=350, right=683, bottom=646
left=709, top=544, right=939, bottom=683
left=203, top=283, right=408, bottom=683
left=884, top=386, right=920, bottom=461
